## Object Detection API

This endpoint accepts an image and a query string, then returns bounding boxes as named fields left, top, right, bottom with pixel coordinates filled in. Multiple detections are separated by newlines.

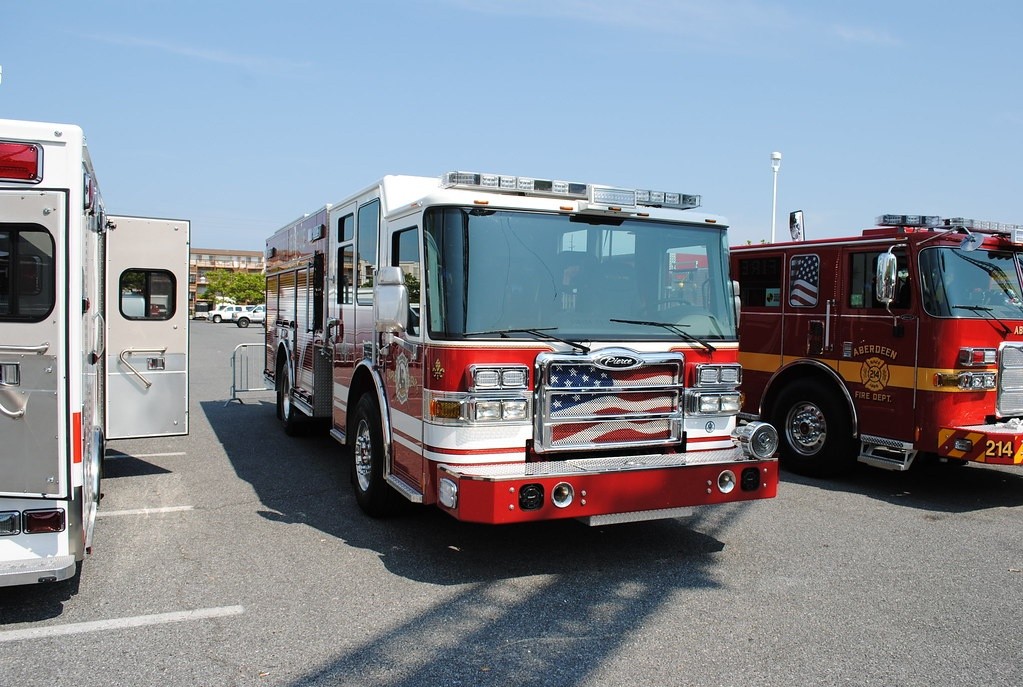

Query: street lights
left=770, top=151, right=782, bottom=242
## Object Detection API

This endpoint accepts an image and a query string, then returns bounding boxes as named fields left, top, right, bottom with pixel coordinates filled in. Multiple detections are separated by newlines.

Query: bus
left=193, top=302, right=209, bottom=320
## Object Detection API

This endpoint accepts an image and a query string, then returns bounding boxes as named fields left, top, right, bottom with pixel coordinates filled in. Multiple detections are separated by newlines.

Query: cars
left=231, top=304, right=265, bottom=328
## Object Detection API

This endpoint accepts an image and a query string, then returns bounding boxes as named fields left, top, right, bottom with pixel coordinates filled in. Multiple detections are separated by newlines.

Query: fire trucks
left=0, top=119, right=190, bottom=588
left=564, top=212, right=1023, bottom=476
left=265, top=169, right=778, bottom=525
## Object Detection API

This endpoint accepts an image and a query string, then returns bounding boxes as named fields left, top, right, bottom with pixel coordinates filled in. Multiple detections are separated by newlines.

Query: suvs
left=150, top=304, right=167, bottom=318
left=208, top=304, right=255, bottom=323
